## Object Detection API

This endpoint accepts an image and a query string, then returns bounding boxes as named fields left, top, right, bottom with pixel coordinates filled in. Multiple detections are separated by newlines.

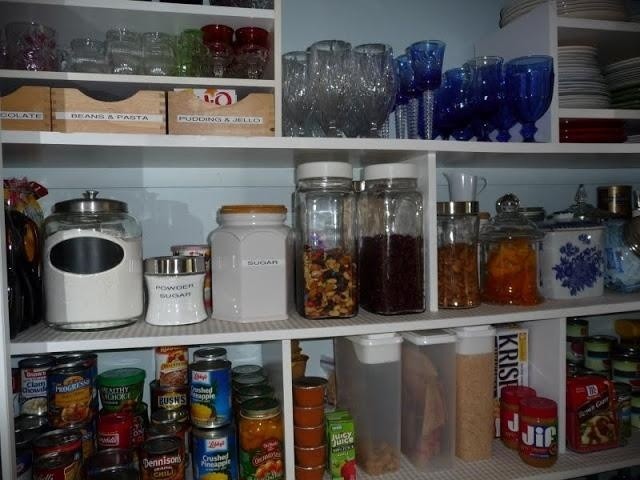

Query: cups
left=0, top=18, right=273, bottom=80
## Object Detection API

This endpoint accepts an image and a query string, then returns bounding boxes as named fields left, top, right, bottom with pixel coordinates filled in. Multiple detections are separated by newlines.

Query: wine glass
left=278, top=40, right=555, bottom=144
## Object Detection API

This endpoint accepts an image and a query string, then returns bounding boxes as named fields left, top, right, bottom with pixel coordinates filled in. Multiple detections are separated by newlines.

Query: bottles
left=437, top=170, right=640, bottom=312
left=44, top=189, right=145, bottom=333
left=359, top=163, right=425, bottom=318
left=6, top=205, right=43, bottom=336
left=208, top=203, right=296, bottom=324
left=139, top=253, right=209, bottom=326
left=169, top=244, right=214, bottom=311
left=292, top=160, right=360, bottom=320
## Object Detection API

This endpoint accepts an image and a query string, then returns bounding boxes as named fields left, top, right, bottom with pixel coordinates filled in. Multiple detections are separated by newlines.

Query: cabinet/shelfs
left=1, top=1, right=639, bottom=480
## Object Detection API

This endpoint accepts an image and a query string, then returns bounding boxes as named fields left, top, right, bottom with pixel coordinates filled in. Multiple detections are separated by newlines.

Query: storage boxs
left=0, top=80, right=279, bottom=139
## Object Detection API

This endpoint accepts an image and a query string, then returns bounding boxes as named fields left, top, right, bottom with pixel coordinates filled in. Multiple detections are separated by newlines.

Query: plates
left=499, top=0, right=629, bottom=30
left=560, top=118, right=640, bottom=143
left=558, top=45, right=640, bottom=109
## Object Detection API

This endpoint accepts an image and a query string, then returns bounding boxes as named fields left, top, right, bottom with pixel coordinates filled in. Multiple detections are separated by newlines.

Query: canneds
left=597, top=185, right=632, bottom=221
left=11, top=343, right=286, bottom=479
left=519, top=397, right=558, bottom=468
left=566, top=317, right=640, bottom=454
left=500, top=386, right=536, bottom=449
